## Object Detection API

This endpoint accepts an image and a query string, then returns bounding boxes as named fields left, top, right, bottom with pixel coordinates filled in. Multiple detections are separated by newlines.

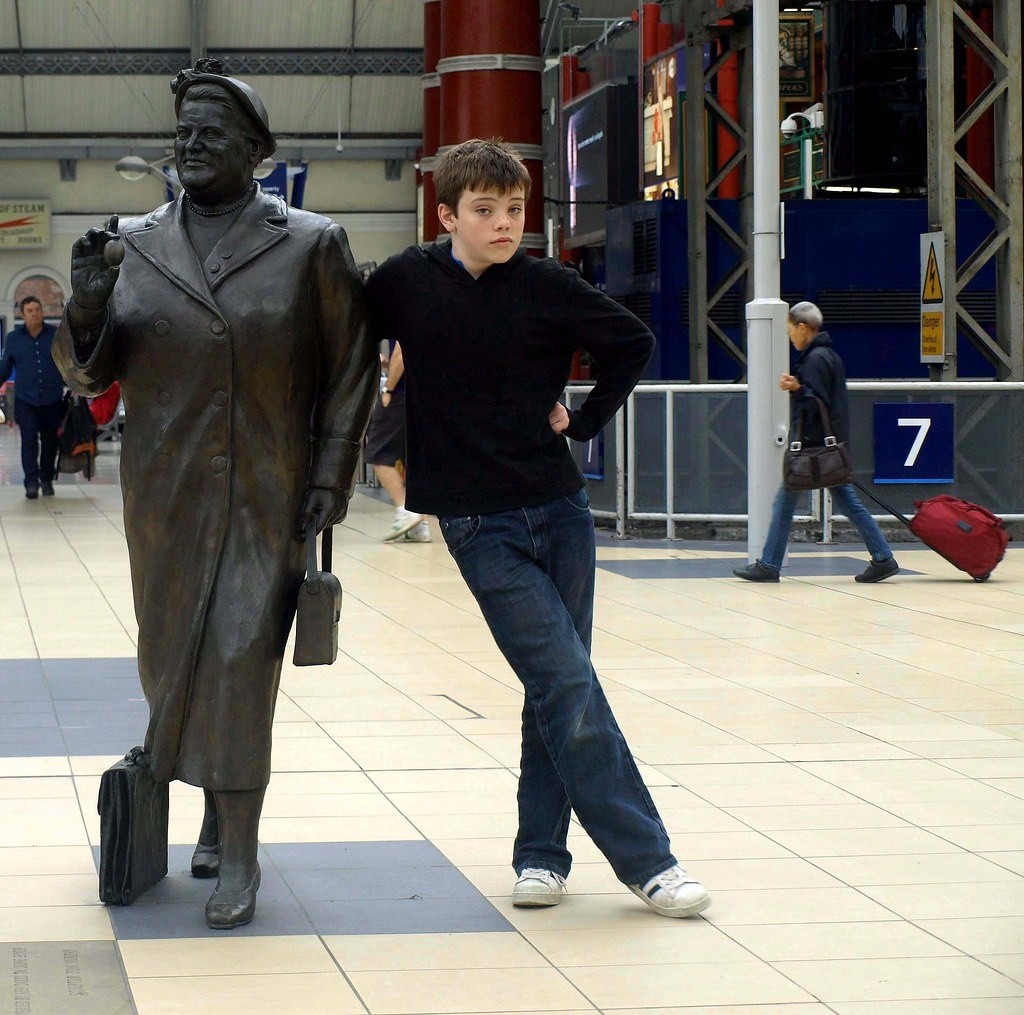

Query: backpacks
left=57, top=388, right=98, bottom=482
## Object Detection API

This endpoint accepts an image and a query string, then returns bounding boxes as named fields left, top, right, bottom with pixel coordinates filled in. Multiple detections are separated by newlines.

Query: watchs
left=382, top=387, right=393, bottom=394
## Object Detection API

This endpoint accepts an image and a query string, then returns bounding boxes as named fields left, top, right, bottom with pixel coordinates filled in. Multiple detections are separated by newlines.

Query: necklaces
left=186, top=185, right=252, bottom=216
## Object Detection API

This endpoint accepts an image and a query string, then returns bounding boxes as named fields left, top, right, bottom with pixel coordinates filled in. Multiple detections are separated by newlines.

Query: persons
left=51, top=58, right=380, bottom=930
left=361, top=135, right=711, bottom=917
left=0, top=296, right=63, bottom=499
left=732, top=302, right=900, bottom=583
left=363, top=340, right=432, bottom=543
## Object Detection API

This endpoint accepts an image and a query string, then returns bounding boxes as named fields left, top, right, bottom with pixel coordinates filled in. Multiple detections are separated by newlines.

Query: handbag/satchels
left=782, top=396, right=853, bottom=490
left=97, top=745, right=169, bottom=905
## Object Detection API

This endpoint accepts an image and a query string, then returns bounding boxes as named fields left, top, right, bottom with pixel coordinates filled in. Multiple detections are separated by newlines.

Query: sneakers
left=625, top=865, right=710, bottom=918
left=383, top=511, right=422, bottom=540
left=732, top=558, right=780, bottom=583
left=386, top=521, right=431, bottom=542
left=856, top=557, right=899, bottom=583
left=512, top=867, right=567, bottom=906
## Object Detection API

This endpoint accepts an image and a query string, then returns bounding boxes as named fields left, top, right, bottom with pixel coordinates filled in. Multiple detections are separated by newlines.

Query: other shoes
left=26, top=485, right=38, bottom=498
left=39, top=480, right=54, bottom=496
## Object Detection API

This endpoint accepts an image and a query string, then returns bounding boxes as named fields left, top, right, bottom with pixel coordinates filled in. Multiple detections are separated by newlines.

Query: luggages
left=851, top=478, right=1012, bottom=583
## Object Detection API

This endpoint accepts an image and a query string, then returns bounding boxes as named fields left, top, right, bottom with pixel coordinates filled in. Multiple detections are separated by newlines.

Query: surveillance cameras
left=780, top=119, right=797, bottom=140
